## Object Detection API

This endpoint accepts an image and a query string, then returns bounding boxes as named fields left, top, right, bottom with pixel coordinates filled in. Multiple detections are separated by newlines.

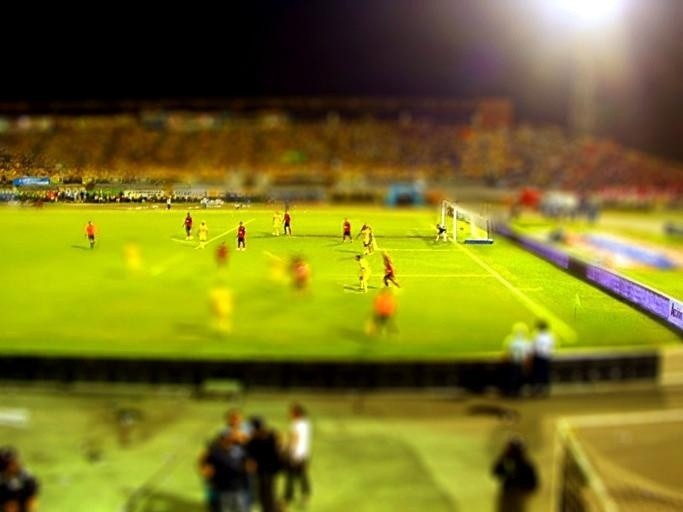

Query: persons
left=84, top=220, right=95, bottom=248
left=506, top=322, right=530, bottom=396
left=291, top=257, right=309, bottom=291
left=210, top=280, right=230, bottom=334
left=492, top=434, right=539, bottom=512
left=529, top=320, right=554, bottom=386
left=183, top=213, right=208, bottom=247
left=435, top=224, right=448, bottom=242
left=368, top=288, right=398, bottom=334
left=0, top=447, right=39, bottom=511
left=0, top=160, right=250, bottom=210
left=236, top=222, right=246, bottom=249
left=272, top=211, right=291, bottom=237
left=215, top=241, right=229, bottom=268
left=198, top=402, right=312, bottom=512
left=342, top=217, right=400, bottom=294
left=508, top=186, right=597, bottom=245
